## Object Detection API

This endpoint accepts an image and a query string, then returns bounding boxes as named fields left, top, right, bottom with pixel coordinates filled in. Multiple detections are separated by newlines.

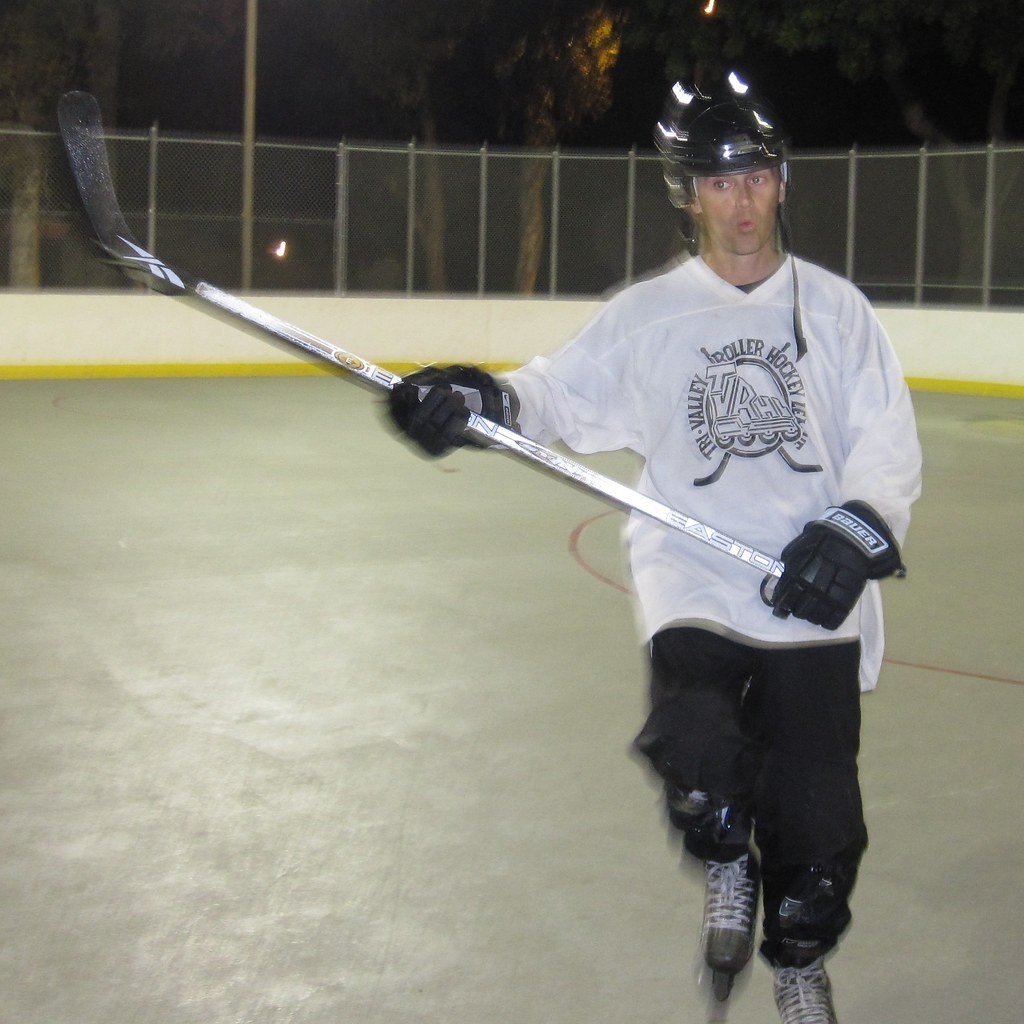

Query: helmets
left=652, top=66, right=788, bottom=189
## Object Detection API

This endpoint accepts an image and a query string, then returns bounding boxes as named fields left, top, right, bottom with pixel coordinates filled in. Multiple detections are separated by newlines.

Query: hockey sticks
left=55, top=83, right=788, bottom=580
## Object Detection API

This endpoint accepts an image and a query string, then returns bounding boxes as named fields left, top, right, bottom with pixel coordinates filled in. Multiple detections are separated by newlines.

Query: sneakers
left=775, top=954, right=836, bottom=1024
left=702, top=847, right=758, bottom=1000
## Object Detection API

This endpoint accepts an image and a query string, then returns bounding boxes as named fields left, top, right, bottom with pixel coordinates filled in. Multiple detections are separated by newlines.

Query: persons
left=388, top=69, right=923, bottom=1024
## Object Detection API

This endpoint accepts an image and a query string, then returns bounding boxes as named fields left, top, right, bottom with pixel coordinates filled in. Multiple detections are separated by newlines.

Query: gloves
left=390, top=364, right=512, bottom=457
left=760, top=502, right=904, bottom=630
